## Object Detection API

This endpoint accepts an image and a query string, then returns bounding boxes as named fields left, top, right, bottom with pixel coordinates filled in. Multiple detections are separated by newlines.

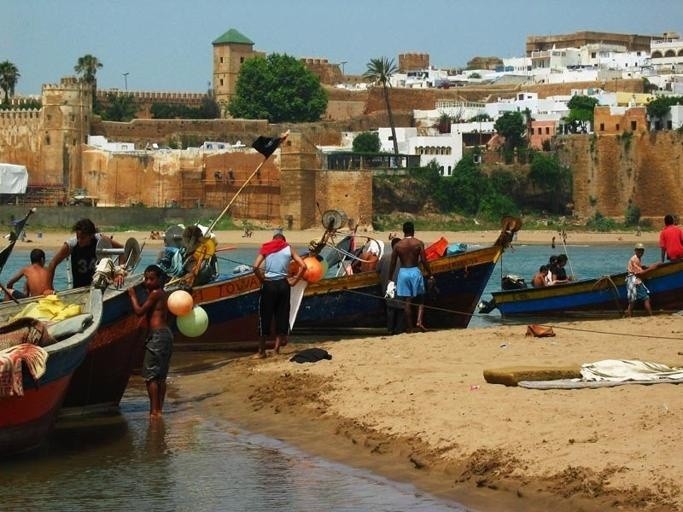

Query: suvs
left=68, top=188, right=100, bottom=206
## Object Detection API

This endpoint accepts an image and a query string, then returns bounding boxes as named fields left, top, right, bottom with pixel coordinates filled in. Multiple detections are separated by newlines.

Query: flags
left=251, top=130, right=288, bottom=161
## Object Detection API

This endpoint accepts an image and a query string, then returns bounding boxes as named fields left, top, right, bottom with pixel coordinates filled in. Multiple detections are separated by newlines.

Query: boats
left=476, top=255, right=682, bottom=322
left=292, top=214, right=523, bottom=336
left=60, top=264, right=151, bottom=432
left=0, top=256, right=121, bottom=458
left=166, top=201, right=349, bottom=349
left=0, top=204, right=36, bottom=287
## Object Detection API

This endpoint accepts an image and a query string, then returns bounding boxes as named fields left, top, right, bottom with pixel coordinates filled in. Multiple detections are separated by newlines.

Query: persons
left=4, top=250, right=50, bottom=301
left=388, top=222, right=435, bottom=334
left=154, top=231, right=159, bottom=238
left=244, top=226, right=249, bottom=237
left=530, top=265, right=547, bottom=289
left=128, top=265, right=170, bottom=416
left=376, top=238, right=409, bottom=334
left=551, top=255, right=570, bottom=284
left=656, top=215, right=682, bottom=262
left=42, top=219, right=124, bottom=287
left=250, top=230, right=306, bottom=359
left=150, top=231, right=154, bottom=239
left=542, top=256, right=557, bottom=286
left=624, top=244, right=653, bottom=317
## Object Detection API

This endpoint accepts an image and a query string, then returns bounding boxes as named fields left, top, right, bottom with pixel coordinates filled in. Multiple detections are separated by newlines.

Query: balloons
left=176, top=307, right=208, bottom=337
left=167, top=290, right=192, bottom=317
left=301, top=256, right=322, bottom=282
left=315, top=255, right=329, bottom=278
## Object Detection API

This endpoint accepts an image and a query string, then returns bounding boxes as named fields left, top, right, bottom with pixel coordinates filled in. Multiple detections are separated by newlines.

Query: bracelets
left=428, top=275, right=434, bottom=279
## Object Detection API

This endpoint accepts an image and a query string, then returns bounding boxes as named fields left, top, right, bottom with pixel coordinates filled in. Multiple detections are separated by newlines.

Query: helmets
left=634, top=243, right=644, bottom=250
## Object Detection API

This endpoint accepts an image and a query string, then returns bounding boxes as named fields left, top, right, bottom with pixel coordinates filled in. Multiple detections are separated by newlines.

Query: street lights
left=119, top=72, right=130, bottom=90
left=340, top=60, right=347, bottom=75
